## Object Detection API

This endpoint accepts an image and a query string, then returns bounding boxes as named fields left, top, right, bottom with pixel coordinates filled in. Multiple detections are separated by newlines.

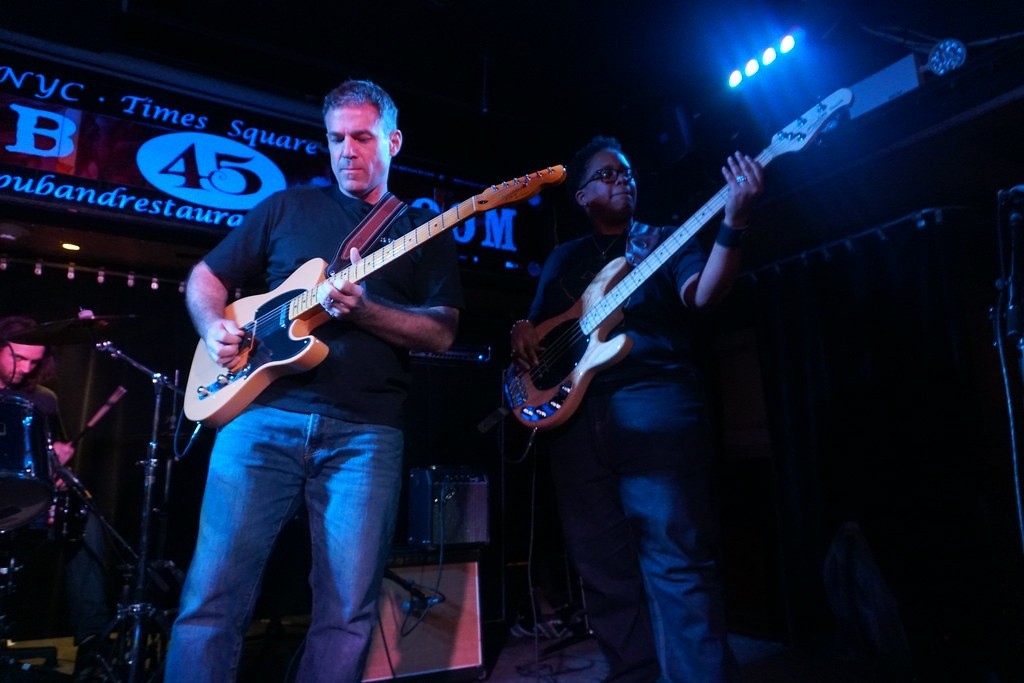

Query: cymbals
left=6, top=314, right=142, bottom=346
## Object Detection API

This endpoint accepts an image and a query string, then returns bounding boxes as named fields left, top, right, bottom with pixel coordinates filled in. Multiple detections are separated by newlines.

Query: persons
left=0, top=317, right=151, bottom=683
left=162, top=79, right=463, bottom=683
left=509, top=134, right=763, bottom=683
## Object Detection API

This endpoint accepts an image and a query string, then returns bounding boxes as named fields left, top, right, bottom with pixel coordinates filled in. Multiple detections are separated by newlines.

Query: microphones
left=400, top=597, right=443, bottom=613
left=997, top=183, right=1024, bottom=206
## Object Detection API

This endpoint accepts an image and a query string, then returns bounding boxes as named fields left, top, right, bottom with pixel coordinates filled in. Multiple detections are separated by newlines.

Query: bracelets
left=510, top=319, right=530, bottom=334
left=716, top=219, right=750, bottom=252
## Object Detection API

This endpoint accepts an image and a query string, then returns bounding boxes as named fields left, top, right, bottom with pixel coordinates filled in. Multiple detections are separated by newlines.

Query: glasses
left=579, top=167, right=640, bottom=189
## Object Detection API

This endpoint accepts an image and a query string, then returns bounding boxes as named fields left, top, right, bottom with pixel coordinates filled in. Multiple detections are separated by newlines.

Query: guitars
left=182, top=164, right=567, bottom=428
left=501, top=87, right=855, bottom=430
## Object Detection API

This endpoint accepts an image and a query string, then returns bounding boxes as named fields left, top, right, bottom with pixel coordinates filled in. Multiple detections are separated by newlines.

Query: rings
left=330, top=299, right=334, bottom=304
left=512, top=349, right=516, bottom=353
left=736, top=176, right=746, bottom=183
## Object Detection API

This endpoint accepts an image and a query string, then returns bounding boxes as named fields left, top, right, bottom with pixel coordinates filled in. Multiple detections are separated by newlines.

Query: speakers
left=356, top=465, right=490, bottom=683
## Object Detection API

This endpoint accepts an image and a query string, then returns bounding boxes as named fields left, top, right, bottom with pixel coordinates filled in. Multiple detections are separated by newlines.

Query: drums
left=0, top=393, right=54, bottom=506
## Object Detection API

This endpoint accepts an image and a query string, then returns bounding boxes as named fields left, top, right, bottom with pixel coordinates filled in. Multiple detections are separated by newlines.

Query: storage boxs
left=360, top=552, right=486, bottom=682
left=408, top=464, right=491, bottom=550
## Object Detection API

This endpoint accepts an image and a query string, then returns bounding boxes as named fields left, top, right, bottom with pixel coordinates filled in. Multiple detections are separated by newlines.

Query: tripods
left=73, top=338, right=187, bottom=682
